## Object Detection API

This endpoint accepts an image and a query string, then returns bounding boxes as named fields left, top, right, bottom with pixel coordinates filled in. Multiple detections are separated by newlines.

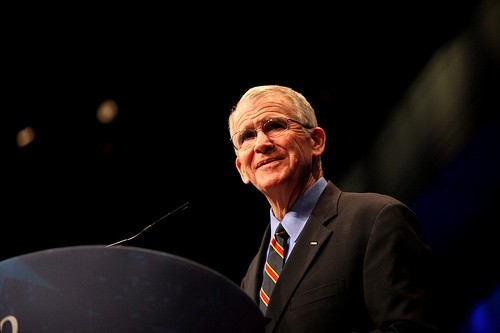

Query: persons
left=228, top=85, right=438, bottom=333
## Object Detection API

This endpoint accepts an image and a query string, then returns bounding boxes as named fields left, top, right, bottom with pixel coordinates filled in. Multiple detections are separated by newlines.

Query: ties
left=258, top=228, right=291, bottom=317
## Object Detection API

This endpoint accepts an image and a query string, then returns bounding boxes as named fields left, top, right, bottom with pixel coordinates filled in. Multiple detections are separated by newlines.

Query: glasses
left=230, top=117, right=313, bottom=150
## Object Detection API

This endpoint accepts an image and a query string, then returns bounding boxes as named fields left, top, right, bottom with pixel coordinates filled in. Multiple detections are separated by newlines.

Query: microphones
left=108, top=202, right=190, bottom=247
